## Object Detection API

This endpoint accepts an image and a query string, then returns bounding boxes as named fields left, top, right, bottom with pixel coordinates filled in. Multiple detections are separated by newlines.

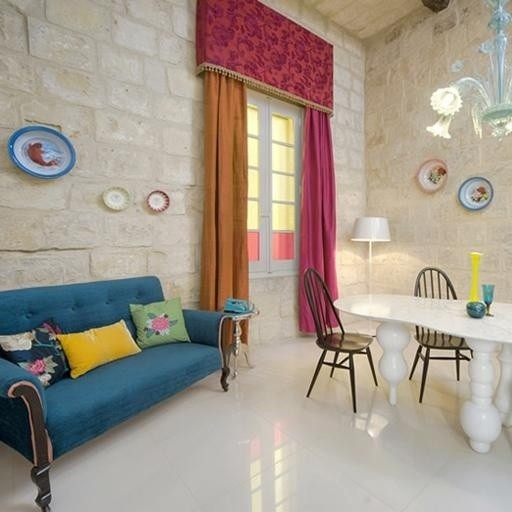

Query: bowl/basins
left=458, top=176, right=494, bottom=211
left=7, top=125, right=76, bottom=179
left=418, top=158, right=449, bottom=193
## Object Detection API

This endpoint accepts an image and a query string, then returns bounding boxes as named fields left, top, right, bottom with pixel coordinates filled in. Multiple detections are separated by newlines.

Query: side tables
left=220, top=308, right=260, bottom=379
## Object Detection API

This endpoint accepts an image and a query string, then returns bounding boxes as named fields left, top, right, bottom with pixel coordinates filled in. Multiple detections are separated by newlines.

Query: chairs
left=408, top=267, right=473, bottom=403
left=302, top=266, right=379, bottom=414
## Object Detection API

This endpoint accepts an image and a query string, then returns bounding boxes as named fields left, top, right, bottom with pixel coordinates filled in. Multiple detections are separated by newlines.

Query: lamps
left=351, top=217, right=393, bottom=293
left=425, top=0, right=512, bottom=140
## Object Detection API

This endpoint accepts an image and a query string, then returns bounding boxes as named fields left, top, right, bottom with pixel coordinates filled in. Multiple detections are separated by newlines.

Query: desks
left=333, top=292, right=512, bottom=455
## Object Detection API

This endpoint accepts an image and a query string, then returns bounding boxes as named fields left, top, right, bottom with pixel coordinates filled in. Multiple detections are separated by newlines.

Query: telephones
left=224, top=299, right=249, bottom=313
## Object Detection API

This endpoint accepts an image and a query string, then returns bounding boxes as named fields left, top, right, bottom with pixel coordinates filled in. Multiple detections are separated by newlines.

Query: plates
left=103, top=185, right=132, bottom=210
left=147, top=190, right=169, bottom=212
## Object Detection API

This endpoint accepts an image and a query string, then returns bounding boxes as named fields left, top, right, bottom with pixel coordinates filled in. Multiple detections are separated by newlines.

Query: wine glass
left=482, top=284, right=494, bottom=316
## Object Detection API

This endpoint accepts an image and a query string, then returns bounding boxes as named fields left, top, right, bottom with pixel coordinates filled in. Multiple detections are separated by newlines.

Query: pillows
left=0, top=297, right=191, bottom=389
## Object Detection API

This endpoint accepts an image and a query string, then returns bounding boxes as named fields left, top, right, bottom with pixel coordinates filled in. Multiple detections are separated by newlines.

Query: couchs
left=0, top=276, right=233, bottom=512
